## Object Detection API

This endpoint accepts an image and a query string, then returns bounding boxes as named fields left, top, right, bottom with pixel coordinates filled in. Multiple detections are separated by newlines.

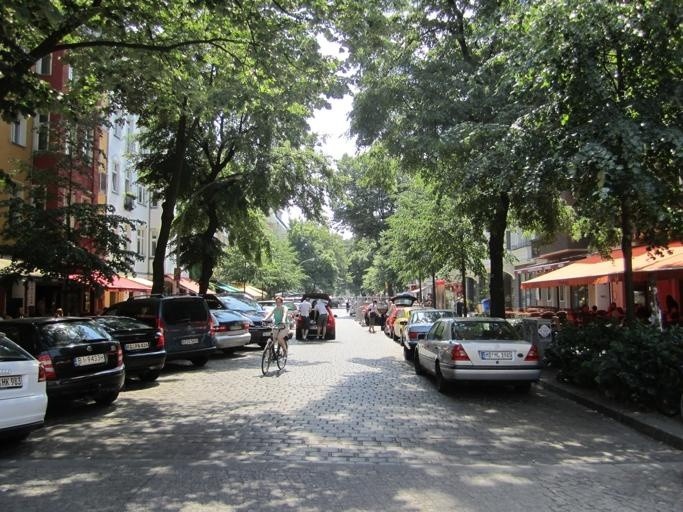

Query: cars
left=0, top=332, right=48, bottom=442
left=365, top=294, right=541, bottom=393
left=101, top=291, right=335, bottom=371
left=0, top=314, right=126, bottom=417
left=86, top=314, right=165, bottom=389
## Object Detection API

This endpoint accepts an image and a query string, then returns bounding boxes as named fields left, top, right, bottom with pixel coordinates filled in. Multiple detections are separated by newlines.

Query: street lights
left=176, top=173, right=245, bottom=296
left=297, top=258, right=315, bottom=266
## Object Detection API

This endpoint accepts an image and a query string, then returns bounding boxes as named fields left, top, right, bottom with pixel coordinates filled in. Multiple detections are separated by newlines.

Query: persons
left=37, top=297, right=46, bottom=316
left=457, top=298, right=464, bottom=317
left=385, top=300, right=397, bottom=328
left=53, top=308, right=64, bottom=317
left=365, top=300, right=380, bottom=333
left=575, top=294, right=683, bottom=327
left=0, top=306, right=39, bottom=320
left=263, top=296, right=289, bottom=360
left=300, top=297, right=328, bottom=340
left=425, top=293, right=434, bottom=307
left=346, top=298, right=355, bottom=312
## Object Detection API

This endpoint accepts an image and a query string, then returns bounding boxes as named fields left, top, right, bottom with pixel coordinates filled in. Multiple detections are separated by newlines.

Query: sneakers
left=301, top=338, right=309, bottom=342
left=316, top=336, right=325, bottom=339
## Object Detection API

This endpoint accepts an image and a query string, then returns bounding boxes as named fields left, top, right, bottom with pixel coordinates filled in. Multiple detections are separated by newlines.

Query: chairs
left=510, top=306, right=628, bottom=328
